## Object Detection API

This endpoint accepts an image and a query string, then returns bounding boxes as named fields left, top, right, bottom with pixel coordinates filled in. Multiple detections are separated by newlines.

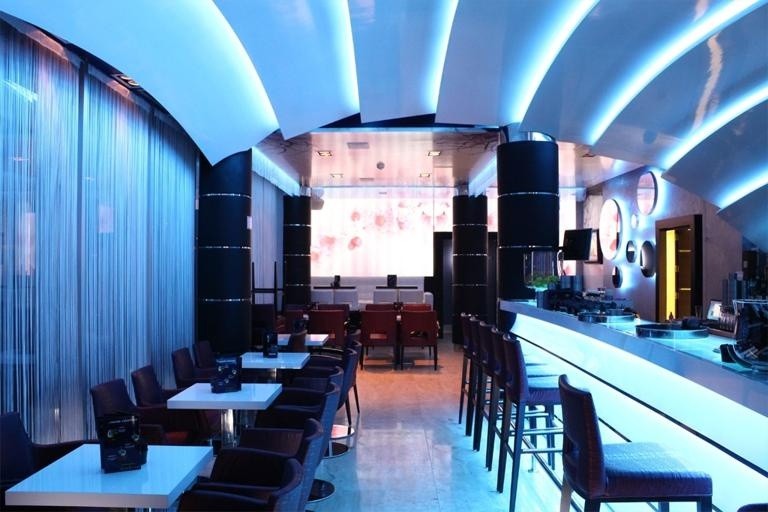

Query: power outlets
left=247, top=216, right=253, bottom=229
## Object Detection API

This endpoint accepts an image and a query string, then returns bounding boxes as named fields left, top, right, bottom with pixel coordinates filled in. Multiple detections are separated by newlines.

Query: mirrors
left=612, top=266, right=622, bottom=288
left=626, top=240, right=637, bottom=263
left=639, top=240, right=656, bottom=277
left=637, top=171, right=658, bottom=216
left=631, top=215, right=639, bottom=228
left=598, top=199, right=623, bottom=261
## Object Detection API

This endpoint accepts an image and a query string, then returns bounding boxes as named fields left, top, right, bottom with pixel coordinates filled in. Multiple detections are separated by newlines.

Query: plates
left=576, top=312, right=634, bottom=323
left=635, top=322, right=708, bottom=340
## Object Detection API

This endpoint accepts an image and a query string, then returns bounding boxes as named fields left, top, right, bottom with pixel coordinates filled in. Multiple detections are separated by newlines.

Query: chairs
left=90, top=379, right=191, bottom=446
left=467, top=317, right=480, bottom=450
left=287, top=304, right=310, bottom=314
left=499, top=335, right=561, bottom=512
left=319, top=304, right=350, bottom=321
left=334, top=289, right=358, bottom=308
left=360, top=311, right=398, bottom=370
left=560, top=374, right=713, bottom=512
left=0, top=412, right=100, bottom=512
left=488, top=327, right=558, bottom=491
left=424, top=292, right=433, bottom=311
left=131, top=366, right=221, bottom=455
left=277, top=310, right=303, bottom=333
left=211, top=418, right=325, bottom=512
left=307, top=309, right=346, bottom=349
left=399, top=311, right=437, bottom=370
left=176, top=458, right=305, bottom=512
left=458, top=312, right=471, bottom=429
left=365, top=303, right=394, bottom=356
left=254, top=382, right=340, bottom=503
left=398, top=289, right=423, bottom=304
left=402, top=304, right=432, bottom=356
left=319, top=341, right=360, bottom=425
left=373, top=289, right=397, bottom=303
left=475, top=321, right=547, bottom=467
left=192, top=342, right=217, bottom=368
left=306, top=349, right=355, bottom=440
left=240, top=352, right=310, bottom=384
left=311, top=289, right=334, bottom=304
left=172, top=348, right=214, bottom=389
left=282, top=366, right=348, bottom=459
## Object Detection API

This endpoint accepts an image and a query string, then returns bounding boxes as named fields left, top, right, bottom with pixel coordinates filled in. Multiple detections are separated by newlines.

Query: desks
left=167, top=383, right=282, bottom=449
left=5, top=444, right=213, bottom=512
left=267, top=333, right=329, bottom=352
